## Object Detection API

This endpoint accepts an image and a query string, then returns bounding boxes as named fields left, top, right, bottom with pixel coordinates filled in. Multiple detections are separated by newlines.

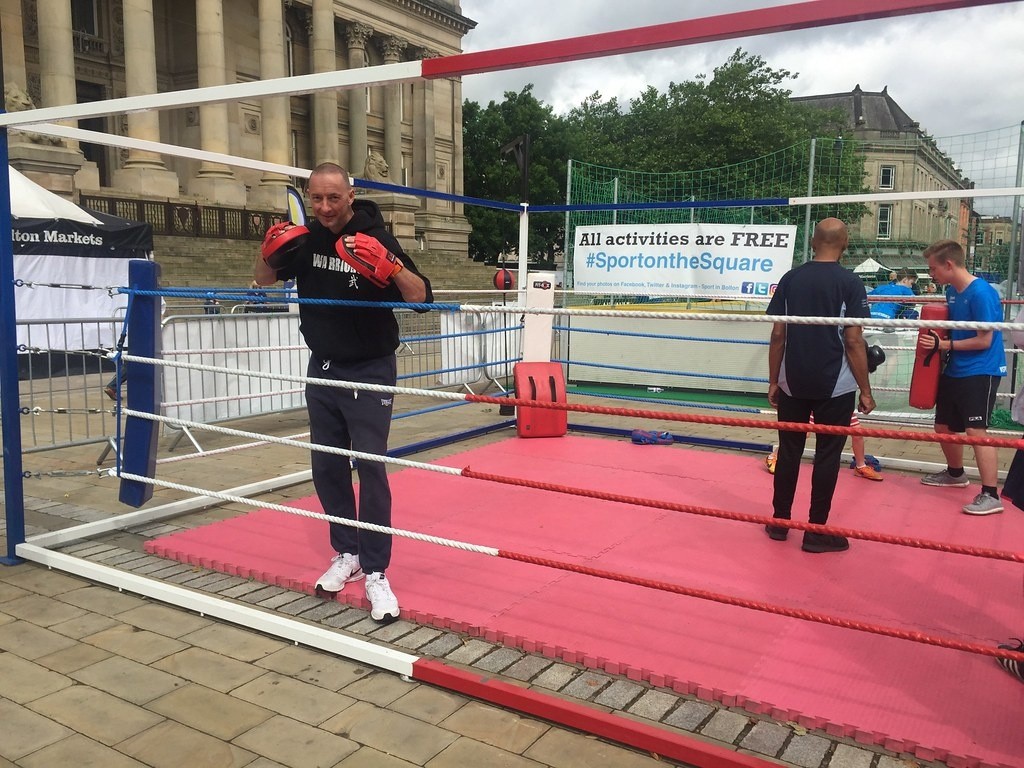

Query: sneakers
left=313, top=551, right=366, bottom=593
left=963, top=494, right=1004, bottom=514
left=364, top=571, right=401, bottom=620
left=765, top=448, right=778, bottom=473
left=921, top=470, right=969, bottom=487
left=994, top=638, right=1024, bottom=682
left=853, top=464, right=884, bottom=480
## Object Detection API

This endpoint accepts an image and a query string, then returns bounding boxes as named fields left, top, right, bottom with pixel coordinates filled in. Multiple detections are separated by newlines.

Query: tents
left=849, top=257, right=895, bottom=279
left=8, top=165, right=156, bottom=355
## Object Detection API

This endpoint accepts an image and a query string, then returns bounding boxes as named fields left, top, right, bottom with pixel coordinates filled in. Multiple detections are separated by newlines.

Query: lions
left=352, top=151, right=391, bottom=194
left=5, top=79, right=64, bottom=143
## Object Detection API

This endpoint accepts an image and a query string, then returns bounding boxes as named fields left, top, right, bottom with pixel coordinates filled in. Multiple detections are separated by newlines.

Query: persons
left=104, top=293, right=166, bottom=400
left=919, top=239, right=1008, bottom=515
left=247, top=280, right=267, bottom=313
left=765, top=217, right=878, bottom=552
left=866, top=266, right=937, bottom=320
left=254, top=162, right=434, bottom=621
left=763, top=340, right=886, bottom=480
left=995, top=306, right=1024, bottom=681
left=203, top=291, right=220, bottom=314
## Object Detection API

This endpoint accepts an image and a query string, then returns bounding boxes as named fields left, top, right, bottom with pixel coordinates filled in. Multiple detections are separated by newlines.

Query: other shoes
left=803, top=530, right=849, bottom=553
left=766, top=518, right=789, bottom=541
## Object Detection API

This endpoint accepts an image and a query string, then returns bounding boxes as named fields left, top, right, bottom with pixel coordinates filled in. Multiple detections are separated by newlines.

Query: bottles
left=647, top=387, right=664, bottom=393
